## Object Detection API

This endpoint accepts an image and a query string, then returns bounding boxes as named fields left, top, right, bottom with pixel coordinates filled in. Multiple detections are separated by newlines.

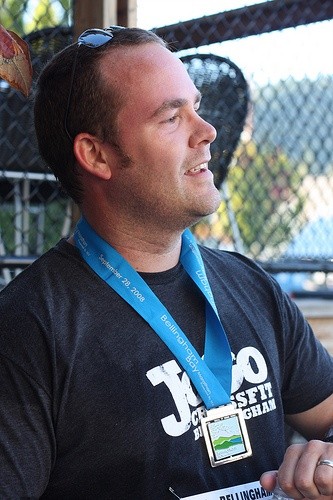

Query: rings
left=317, top=460, right=333, bottom=467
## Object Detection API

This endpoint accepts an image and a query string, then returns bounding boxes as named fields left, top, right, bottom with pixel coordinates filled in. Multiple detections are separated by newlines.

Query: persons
left=0, top=25, right=333, bottom=500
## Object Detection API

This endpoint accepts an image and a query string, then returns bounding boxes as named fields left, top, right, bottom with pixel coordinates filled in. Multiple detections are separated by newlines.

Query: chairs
left=0, top=25, right=73, bottom=288
left=179, top=54, right=252, bottom=255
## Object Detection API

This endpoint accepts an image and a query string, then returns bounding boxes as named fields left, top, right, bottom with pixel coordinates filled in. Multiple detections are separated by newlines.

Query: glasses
left=65, top=25, right=125, bottom=143
